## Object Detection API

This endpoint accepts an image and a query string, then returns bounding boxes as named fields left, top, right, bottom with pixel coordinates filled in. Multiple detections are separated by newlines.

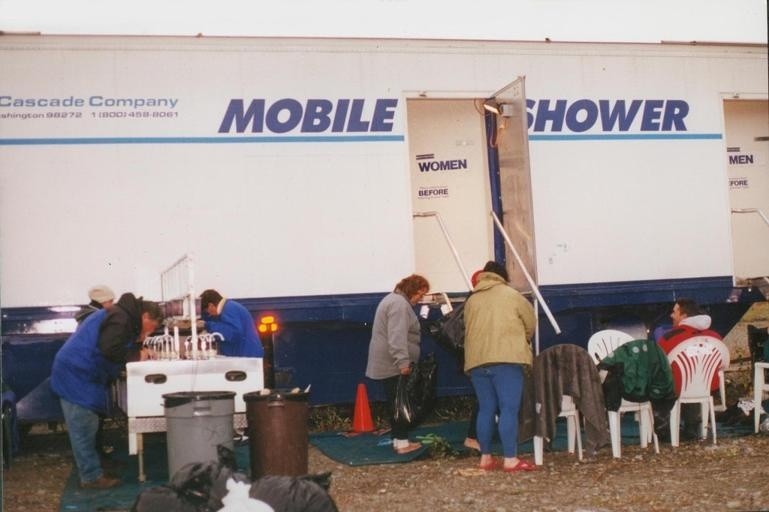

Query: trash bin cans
left=243, top=388, right=311, bottom=484
left=162, top=392, right=237, bottom=489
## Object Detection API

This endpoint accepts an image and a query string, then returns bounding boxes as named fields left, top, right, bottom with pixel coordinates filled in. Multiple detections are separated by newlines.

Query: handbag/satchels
left=391, top=352, right=443, bottom=431
left=428, top=290, right=467, bottom=358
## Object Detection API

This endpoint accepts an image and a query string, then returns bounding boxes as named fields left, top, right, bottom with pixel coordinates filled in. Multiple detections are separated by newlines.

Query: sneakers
left=464, top=437, right=481, bottom=452
left=80, top=475, right=121, bottom=490
left=393, top=442, right=422, bottom=455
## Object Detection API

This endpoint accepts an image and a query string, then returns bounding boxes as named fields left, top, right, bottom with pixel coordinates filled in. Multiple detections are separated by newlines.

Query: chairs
left=588, top=329, right=635, bottom=367
left=665, top=336, right=730, bottom=447
left=529, top=343, right=603, bottom=466
left=598, top=339, right=673, bottom=459
left=753, top=362, right=769, bottom=434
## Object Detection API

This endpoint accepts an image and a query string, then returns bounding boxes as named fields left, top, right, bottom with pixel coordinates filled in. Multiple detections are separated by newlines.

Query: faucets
left=184, top=331, right=224, bottom=361
left=141, top=334, right=175, bottom=361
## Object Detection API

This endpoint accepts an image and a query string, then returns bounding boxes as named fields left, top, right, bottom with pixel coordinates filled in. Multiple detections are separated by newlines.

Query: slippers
left=477, top=457, right=498, bottom=469
left=502, top=457, right=544, bottom=472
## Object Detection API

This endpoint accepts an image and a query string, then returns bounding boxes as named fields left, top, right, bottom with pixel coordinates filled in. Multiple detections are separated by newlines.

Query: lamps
left=483, top=99, right=511, bottom=118
left=258, top=316, right=277, bottom=387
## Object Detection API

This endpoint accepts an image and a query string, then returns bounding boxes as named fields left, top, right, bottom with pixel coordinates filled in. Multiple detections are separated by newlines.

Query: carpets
left=307, top=409, right=768, bottom=467
left=14, top=428, right=252, bottom=511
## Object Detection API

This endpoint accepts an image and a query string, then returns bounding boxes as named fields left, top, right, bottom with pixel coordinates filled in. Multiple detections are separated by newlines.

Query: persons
left=75, top=285, right=115, bottom=455
left=50, top=293, right=164, bottom=490
left=463, top=261, right=537, bottom=472
left=655, top=297, right=724, bottom=442
left=463, top=270, right=484, bottom=452
left=365, top=274, right=430, bottom=454
left=196, top=289, right=264, bottom=358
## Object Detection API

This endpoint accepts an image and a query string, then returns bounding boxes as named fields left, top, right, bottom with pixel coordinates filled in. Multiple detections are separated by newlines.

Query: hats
left=88, top=284, right=115, bottom=304
